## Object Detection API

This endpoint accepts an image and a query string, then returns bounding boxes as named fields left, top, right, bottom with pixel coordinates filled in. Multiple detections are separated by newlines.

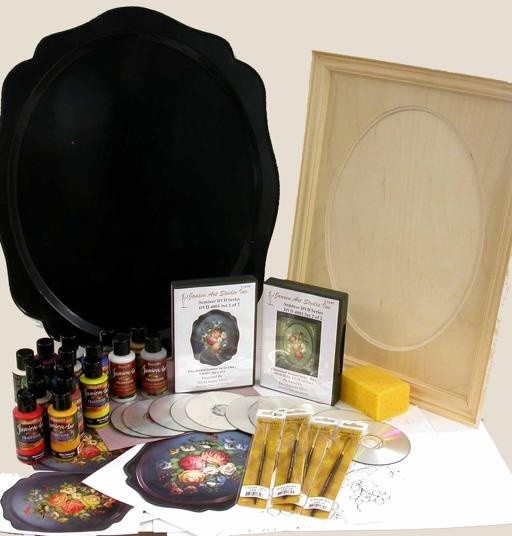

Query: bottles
left=9, top=326, right=170, bottom=464
